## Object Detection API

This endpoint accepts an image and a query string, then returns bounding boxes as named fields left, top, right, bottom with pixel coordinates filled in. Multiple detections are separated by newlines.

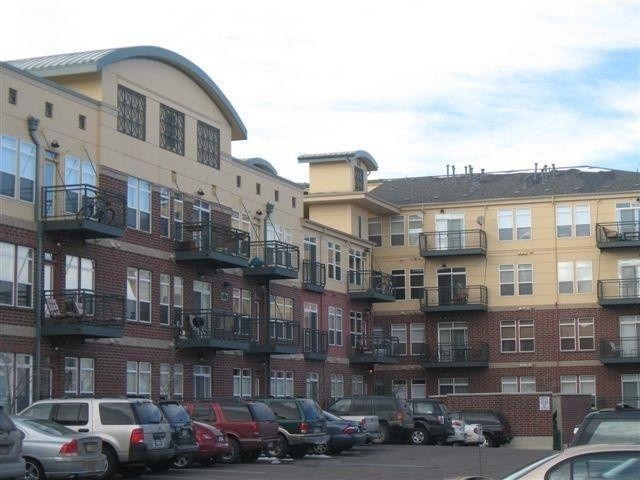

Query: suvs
left=168, top=395, right=331, bottom=464
left=325, top=394, right=514, bottom=447
left=15, top=394, right=200, bottom=480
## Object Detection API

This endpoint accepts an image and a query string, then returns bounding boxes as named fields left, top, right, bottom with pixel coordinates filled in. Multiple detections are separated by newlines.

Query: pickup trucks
left=336, top=415, right=381, bottom=451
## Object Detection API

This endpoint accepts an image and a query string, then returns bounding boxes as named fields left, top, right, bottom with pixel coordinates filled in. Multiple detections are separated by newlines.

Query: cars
left=495, top=408, right=640, bottom=480
left=312, top=408, right=367, bottom=455
left=0, top=397, right=107, bottom=480
left=172, top=419, right=231, bottom=469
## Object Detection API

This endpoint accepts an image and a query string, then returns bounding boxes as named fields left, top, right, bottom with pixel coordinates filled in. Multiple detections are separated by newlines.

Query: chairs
left=607, top=341, right=625, bottom=359
left=603, top=226, right=619, bottom=242
left=71, top=298, right=100, bottom=326
left=45, top=298, right=75, bottom=324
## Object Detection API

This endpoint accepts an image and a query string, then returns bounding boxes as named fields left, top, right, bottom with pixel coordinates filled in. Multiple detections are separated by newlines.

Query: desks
left=624, top=232, right=640, bottom=240
